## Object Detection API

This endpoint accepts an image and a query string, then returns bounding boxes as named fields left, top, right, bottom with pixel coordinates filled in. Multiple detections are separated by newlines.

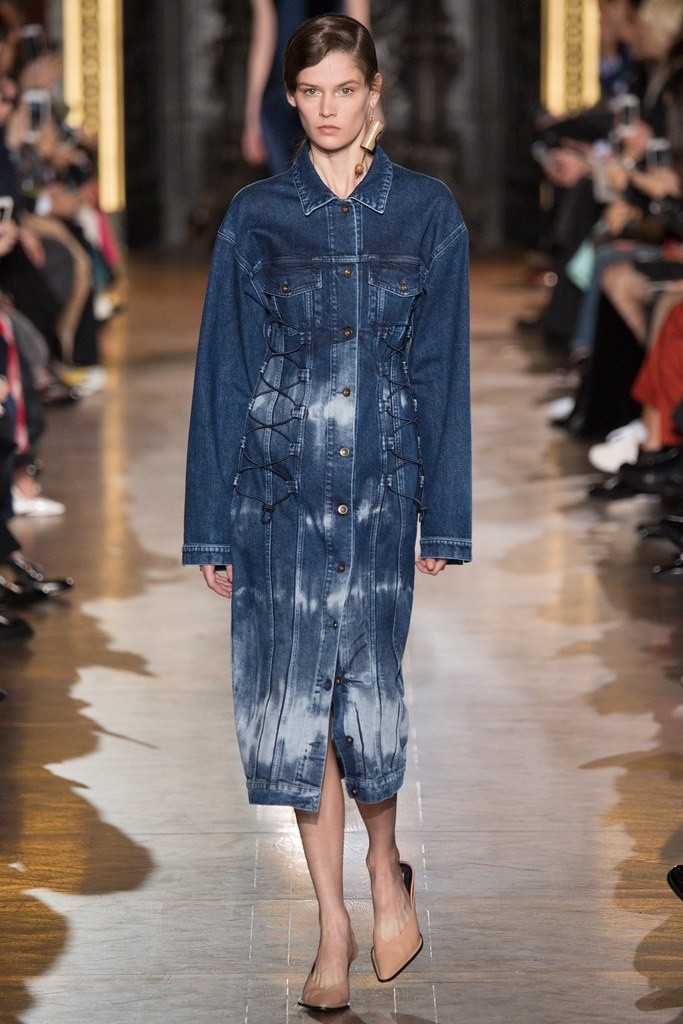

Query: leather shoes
left=372, top=861, right=423, bottom=983
left=297, top=932, right=358, bottom=1009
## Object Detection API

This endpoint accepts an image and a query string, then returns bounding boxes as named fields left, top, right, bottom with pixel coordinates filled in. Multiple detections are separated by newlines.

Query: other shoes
left=589, top=420, right=647, bottom=475
left=591, top=464, right=637, bottom=499
left=0, top=578, right=49, bottom=606
left=12, top=485, right=65, bottom=518
left=1, top=617, right=34, bottom=640
left=10, top=559, right=73, bottom=593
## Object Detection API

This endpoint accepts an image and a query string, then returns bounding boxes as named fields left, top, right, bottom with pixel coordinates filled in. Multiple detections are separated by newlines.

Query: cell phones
left=0, top=195, right=14, bottom=224
left=22, top=87, right=50, bottom=144
left=533, top=143, right=561, bottom=173
left=645, top=139, right=672, bottom=169
left=614, top=93, right=640, bottom=135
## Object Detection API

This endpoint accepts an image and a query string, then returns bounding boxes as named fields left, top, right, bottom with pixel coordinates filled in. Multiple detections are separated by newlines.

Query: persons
left=239, top=0, right=388, bottom=182
left=511, top=0, right=683, bottom=897
left=0, top=0, right=129, bottom=701
left=182, top=13, right=473, bottom=1014
